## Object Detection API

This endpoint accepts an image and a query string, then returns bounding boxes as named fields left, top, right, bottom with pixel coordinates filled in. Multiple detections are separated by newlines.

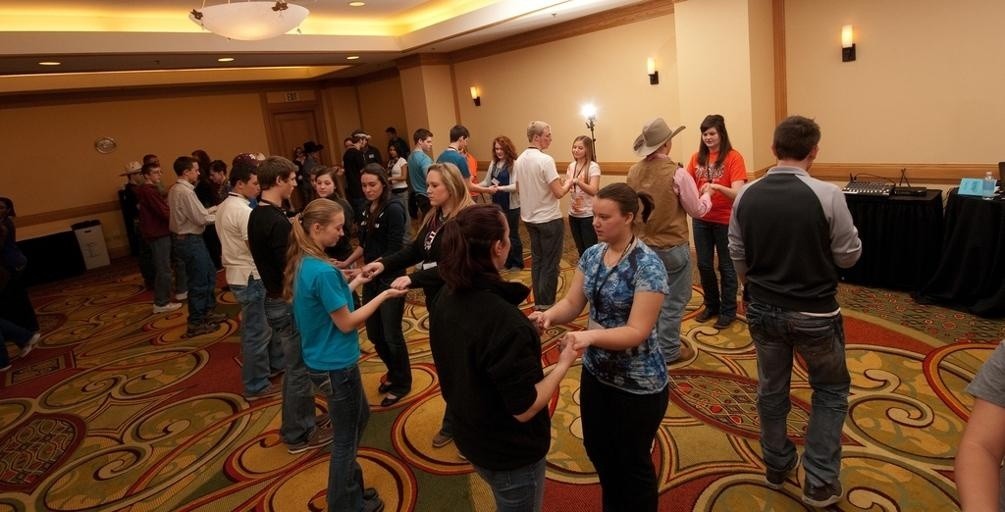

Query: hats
left=117, top=160, right=145, bottom=177
left=632, top=113, right=688, bottom=160
left=298, top=139, right=325, bottom=155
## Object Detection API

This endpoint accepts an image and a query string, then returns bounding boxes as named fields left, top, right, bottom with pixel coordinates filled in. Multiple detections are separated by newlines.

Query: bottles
left=982, top=171, right=996, bottom=201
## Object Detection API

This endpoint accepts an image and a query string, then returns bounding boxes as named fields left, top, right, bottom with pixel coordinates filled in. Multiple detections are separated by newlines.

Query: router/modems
left=892, top=168, right=928, bottom=196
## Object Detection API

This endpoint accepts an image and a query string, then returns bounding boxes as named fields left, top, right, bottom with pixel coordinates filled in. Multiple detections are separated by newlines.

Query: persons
left=954, top=339, right=1005, bottom=512
left=0, top=196, right=41, bottom=371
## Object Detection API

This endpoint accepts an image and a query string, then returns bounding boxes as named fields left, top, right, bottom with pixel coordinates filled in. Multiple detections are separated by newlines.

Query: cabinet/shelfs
left=71, top=218, right=112, bottom=272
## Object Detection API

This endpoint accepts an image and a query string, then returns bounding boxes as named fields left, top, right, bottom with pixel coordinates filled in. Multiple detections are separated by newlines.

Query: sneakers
left=432, top=413, right=469, bottom=460
left=149, top=291, right=230, bottom=339
left=0, top=362, right=12, bottom=372
left=529, top=296, right=556, bottom=313
left=328, top=483, right=385, bottom=512
left=764, top=444, right=801, bottom=487
left=693, top=294, right=733, bottom=330
left=16, top=331, right=42, bottom=360
left=665, top=346, right=696, bottom=365
left=802, top=472, right=845, bottom=505
left=245, top=361, right=413, bottom=458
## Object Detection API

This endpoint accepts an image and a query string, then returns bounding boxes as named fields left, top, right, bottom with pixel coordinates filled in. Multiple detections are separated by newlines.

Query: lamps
left=186, top=0, right=312, bottom=44
left=840, top=19, right=859, bottom=65
left=469, top=86, right=481, bottom=107
left=648, top=56, right=660, bottom=85
left=578, top=100, right=598, bottom=166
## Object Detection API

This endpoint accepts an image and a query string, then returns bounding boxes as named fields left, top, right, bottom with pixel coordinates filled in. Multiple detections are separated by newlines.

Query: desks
left=938, top=187, right=1005, bottom=323
left=15, top=228, right=83, bottom=285
left=838, top=183, right=947, bottom=305
